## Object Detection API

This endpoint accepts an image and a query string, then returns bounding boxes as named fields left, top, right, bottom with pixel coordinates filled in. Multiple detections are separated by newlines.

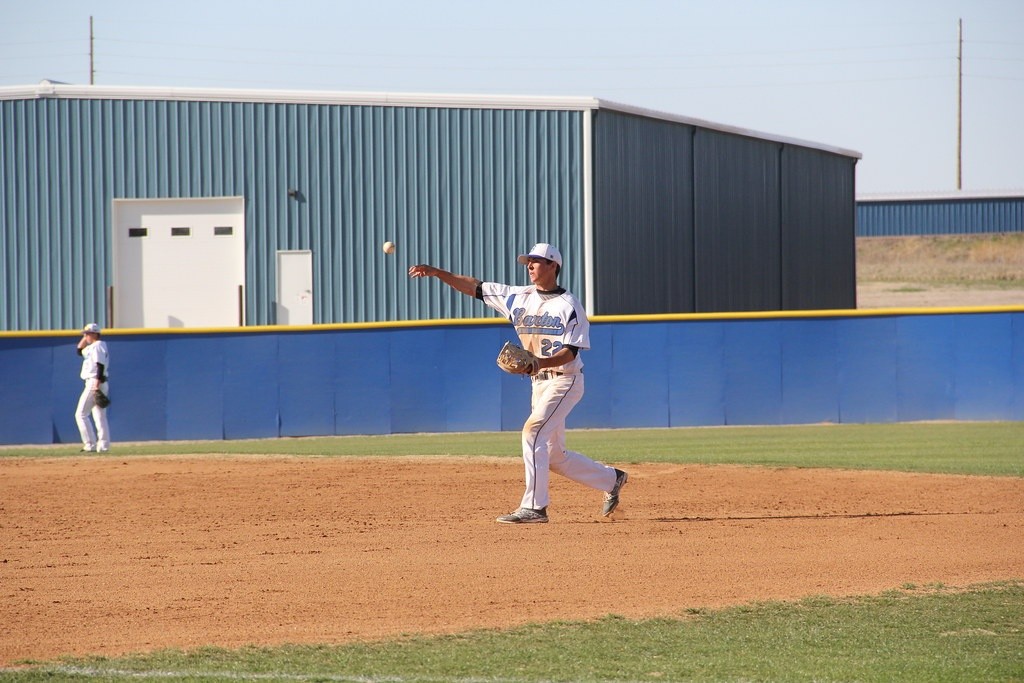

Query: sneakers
left=603, top=469, right=628, bottom=516
left=496, top=507, right=549, bottom=523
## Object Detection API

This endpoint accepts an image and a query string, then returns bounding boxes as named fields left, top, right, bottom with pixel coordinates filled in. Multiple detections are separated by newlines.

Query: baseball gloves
left=94, top=389, right=110, bottom=408
left=496, top=340, right=540, bottom=376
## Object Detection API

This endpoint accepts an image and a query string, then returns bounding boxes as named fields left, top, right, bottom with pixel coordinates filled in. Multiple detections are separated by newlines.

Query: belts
left=535, top=369, right=582, bottom=380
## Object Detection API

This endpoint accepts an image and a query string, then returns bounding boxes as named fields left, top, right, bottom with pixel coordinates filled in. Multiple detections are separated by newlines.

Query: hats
left=517, top=242, right=563, bottom=269
left=82, top=323, right=101, bottom=333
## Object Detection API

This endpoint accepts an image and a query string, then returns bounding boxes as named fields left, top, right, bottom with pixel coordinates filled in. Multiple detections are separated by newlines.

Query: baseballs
left=382, top=241, right=395, bottom=254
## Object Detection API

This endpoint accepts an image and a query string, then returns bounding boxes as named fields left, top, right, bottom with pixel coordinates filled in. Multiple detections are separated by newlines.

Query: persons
left=409, top=244, right=628, bottom=524
left=74, top=324, right=109, bottom=452
